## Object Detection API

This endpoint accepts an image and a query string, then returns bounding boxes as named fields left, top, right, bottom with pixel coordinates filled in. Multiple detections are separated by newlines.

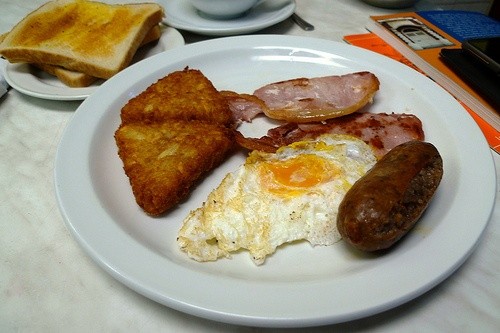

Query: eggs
left=176, top=134, right=378, bottom=266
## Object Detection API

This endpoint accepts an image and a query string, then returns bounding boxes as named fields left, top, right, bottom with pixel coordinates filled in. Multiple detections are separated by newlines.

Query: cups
left=191, top=0, right=267, bottom=18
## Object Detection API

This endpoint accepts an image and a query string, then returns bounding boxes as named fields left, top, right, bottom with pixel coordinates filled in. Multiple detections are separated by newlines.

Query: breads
left=0, top=0, right=166, bottom=89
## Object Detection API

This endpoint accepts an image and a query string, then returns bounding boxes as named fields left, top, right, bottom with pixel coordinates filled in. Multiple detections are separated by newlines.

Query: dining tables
left=0, top=0, right=500, bottom=333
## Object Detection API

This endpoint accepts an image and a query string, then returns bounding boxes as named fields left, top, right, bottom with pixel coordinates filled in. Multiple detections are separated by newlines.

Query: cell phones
left=463, top=36, right=500, bottom=72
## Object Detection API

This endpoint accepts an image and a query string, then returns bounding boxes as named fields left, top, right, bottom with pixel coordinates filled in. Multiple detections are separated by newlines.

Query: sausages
left=337, top=141, right=443, bottom=253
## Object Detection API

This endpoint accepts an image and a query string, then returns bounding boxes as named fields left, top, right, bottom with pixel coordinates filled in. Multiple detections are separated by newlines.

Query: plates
left=147, top=0, right=296, bottom=35
left=53, top=36, right=497, bottom=328
left=2, top=22, right=185, bottom=100
